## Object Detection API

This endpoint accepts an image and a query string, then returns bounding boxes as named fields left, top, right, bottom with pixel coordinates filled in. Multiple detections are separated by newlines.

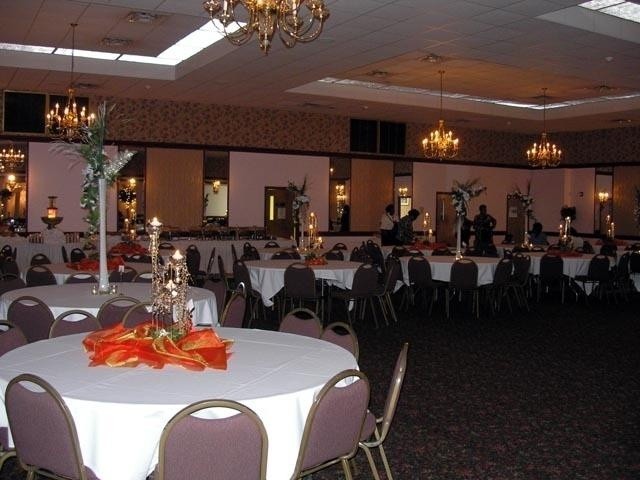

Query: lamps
left=44, top=22, right=97, bottom=142
left=0, top=144, right=25, bottom=175
left=526, top=87, right=562, bottom=170
left=40, top=196, right=64, bottom=230
left=421, top=208, right=433, bottom=249
left=597, top=192, right=609, bottom=232
left=296, top=211, right=323, bottom=266
left=202, top=0, right=331, bottom=58
left=118, top=177, right=137, bottom=231
left=422, top=70, right=459, bottom=161
left=119, top=216, right=195, bottom=347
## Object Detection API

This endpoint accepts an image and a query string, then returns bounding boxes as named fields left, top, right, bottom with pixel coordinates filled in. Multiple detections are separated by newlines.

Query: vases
left=97, top=179, right=111, bottom=294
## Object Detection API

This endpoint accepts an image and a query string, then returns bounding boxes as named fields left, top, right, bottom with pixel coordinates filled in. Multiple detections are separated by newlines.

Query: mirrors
left=116, top=145, right=146, bottom=234
left=202, top=149, right=230, bottom=230
left=393, top=160, right=413, bottom=222
left=0, top=138, right=28, bottom=238
left=594, top=167, right=614, bottom=236
left=329, top=156, right=352, bottom=233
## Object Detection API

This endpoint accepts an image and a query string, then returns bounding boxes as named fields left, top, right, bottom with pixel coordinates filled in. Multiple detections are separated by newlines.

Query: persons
left=473, top=205, right=496, bottom=249
left=567, top=226, right=584, bottom=253
left=398, top=209, right=420, bottom=245
left=530, top=222, right=547, bottom=245
left=379, top=204, right=397, bottom=246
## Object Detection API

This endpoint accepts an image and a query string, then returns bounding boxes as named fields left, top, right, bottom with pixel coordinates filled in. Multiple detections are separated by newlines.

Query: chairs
left=350, top=247, right=363, bottom=262
left=150, top=398, right=269, bottom=480
left=0, top=320, right=28, bottom=359
left=48, top=309, right=102, bottom=339
left=26, top=265, right=57, bottom=287
left=508, top=255, right=531, bottom=313
left=64, top=273, right=98, bottom=283
left=391, top=245, right=407, bottom=256
left=271, top=251, right=291, bottom=260
left=207, top=247, right=218, bottom=281
left=97, top=296, right=147, bottom=329
left=531, top=246, right=544, bottom=252
left=243, top=242, right=251, bottom=254
left=288, top=368, right=370, bottom=480
left=2, top=256, right=19, bottom=276
left=514, top=253, right=523, bottom=269
left=231, top=244, right=239, bottom=262
left=278, top=307, right=323, bottom=339
left=536, top=253, right=570, bottom=316
left=219, top=282, right=247, bottom=329
left=386, top=253, right=410, bottom=312
left=464, top=247, right=476, bottom=256
left=513, top=245, right=530, bottom=252
left=548, top=243, right=567, bottom=252
left=600, top=244, right=617, bottom=273
left=504, top=250, right=513, bottom=259
left=431, top=246, right=451, bottom=256
left=185, top=244, right=206, bottom=280
left=326, top=249, right=344, bottom=261
left=360, top=264, right=398, bottom=327
left=320, top=322, right=359, bottom=362
left=583, top=241, right=594, bottom=254
left=408, top=255, right=445, bottom=317
left=408, top=248, right=423, bottom=255
left=359, top=342, right=409, bottom=480
left=574, top=254, right=609, bottom=293
left=31, top=253, right=51, bottom=266
left=360, top=240, right=367, bottom=255
left=7, top=296, right=53, bottom=341
left=630, top=252, right=640, bottom=273
left=281, top=262, right=324, bottom=322
left=0, top=273, right=25, bottom=297
left=71, top=248, right=86, bottom=262
left=481, top=257, right=513, bottom=318
left=122, top=301, right=174, bottom=332
left=326, top=263, right=379, bottom=334
left=247, top=247, right=261, bottom=261
left=333, top=243, right=347, bottom=250
left=616, top=254, right=629, bottom=286
left=217, top=256, right=234, bottom=279
left=446, top=258, right=480, bottom=324
left=4, top=374, right=100, bottom=480
left=366, top=240, right=384, bottom=271
left=240, top=252, right=256, bottom=262
left=264, top=241, right=280, bottom=248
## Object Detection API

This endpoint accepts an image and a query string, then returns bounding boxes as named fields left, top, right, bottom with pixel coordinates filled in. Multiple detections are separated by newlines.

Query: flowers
left=48, top=101, right=137, bottom=241
